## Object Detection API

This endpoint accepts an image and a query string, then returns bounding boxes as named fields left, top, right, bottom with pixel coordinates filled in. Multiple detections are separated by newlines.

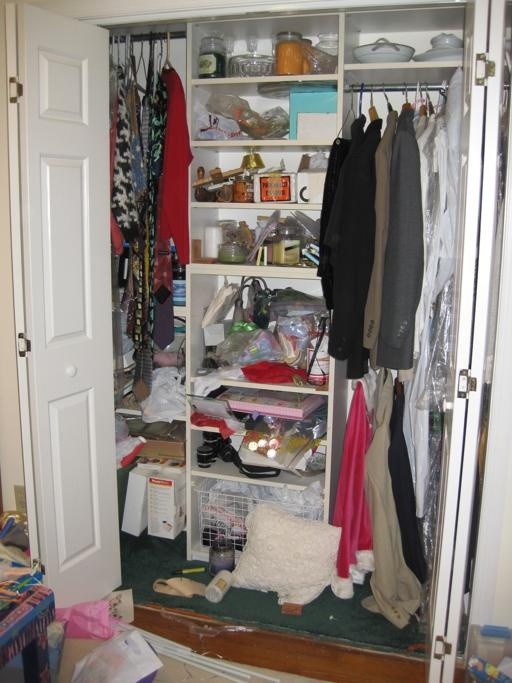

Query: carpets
left=112, top=457, right=426, bottom=660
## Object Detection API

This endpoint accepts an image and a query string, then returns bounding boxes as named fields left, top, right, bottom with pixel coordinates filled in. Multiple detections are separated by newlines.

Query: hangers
left=106, top=28, right=170, bottom=92
left=327, top=84, right=435, bottom=144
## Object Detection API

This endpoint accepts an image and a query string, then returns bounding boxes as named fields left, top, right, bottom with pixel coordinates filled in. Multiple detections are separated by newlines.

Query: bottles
left=196, top=30, right=338, bottom=78
left=170, top=245, right=187, bottom=305
left=120, top=240, right=130, bottom=286
left=204, top=539, right=236, bottom=603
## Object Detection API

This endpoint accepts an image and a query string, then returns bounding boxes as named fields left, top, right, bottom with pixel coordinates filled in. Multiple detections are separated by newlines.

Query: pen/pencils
left=172, top=567, right=205, bottom=574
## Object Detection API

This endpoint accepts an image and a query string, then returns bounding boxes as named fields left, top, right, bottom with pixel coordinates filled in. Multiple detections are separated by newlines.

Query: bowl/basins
left=352, top=33, right=464, bottom=61
left=217, top=243, right=247, bottom=263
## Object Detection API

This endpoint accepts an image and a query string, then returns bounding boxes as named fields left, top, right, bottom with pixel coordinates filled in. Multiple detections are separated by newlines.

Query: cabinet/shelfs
left=77, top=2, right=483, bottom=570
